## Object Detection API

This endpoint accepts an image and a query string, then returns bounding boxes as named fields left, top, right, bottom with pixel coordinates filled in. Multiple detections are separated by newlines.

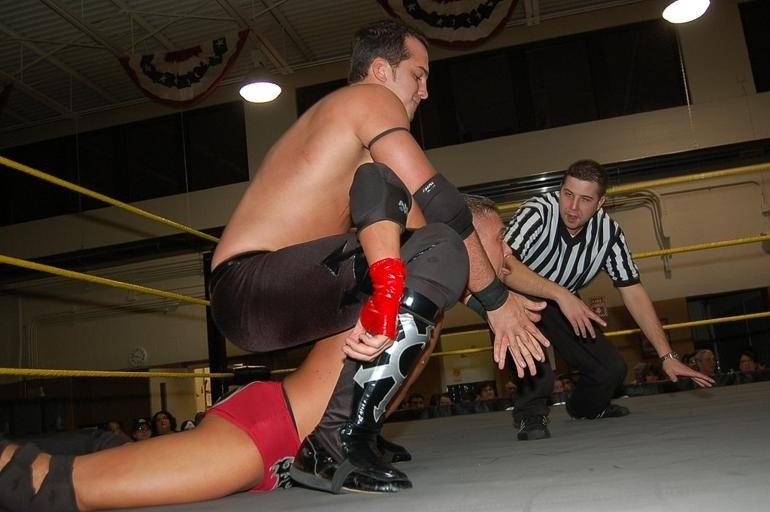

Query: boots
left=288, top=280, right=443, bottom=495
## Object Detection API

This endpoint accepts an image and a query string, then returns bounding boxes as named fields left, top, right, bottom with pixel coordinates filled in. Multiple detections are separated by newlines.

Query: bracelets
left=658, top=351, right=682, bottom=362
left=465, top=293, right=487, bottom=320
left=471, top=274, right=510, bottom=311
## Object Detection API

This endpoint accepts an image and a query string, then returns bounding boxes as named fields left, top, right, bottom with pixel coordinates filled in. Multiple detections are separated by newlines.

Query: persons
left=496, top=158, right=717, bottom=440
left=0, top=158, right=515, bottom=510
left=206, top=17, right=551, bottom=494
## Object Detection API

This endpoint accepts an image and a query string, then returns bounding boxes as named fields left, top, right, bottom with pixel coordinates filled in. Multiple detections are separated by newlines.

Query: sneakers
left=567, top=403, right=630, bottom=421
left=515, top=413, right=552, bottom=441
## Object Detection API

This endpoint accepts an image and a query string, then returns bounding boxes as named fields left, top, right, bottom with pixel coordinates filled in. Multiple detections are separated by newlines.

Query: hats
left=181, top=411, right=206, bottom=430
left=132, top=416, right=151, bottom=427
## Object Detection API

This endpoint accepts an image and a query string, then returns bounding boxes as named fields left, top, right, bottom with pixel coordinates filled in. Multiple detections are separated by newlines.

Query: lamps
left=233, top=57, right=283, bottom=103
left=656, top=0, right=712, bottom=25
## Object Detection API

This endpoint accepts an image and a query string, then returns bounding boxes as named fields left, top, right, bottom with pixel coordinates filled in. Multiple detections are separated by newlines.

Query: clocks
left=127, top=348, right=147, bottom=367
